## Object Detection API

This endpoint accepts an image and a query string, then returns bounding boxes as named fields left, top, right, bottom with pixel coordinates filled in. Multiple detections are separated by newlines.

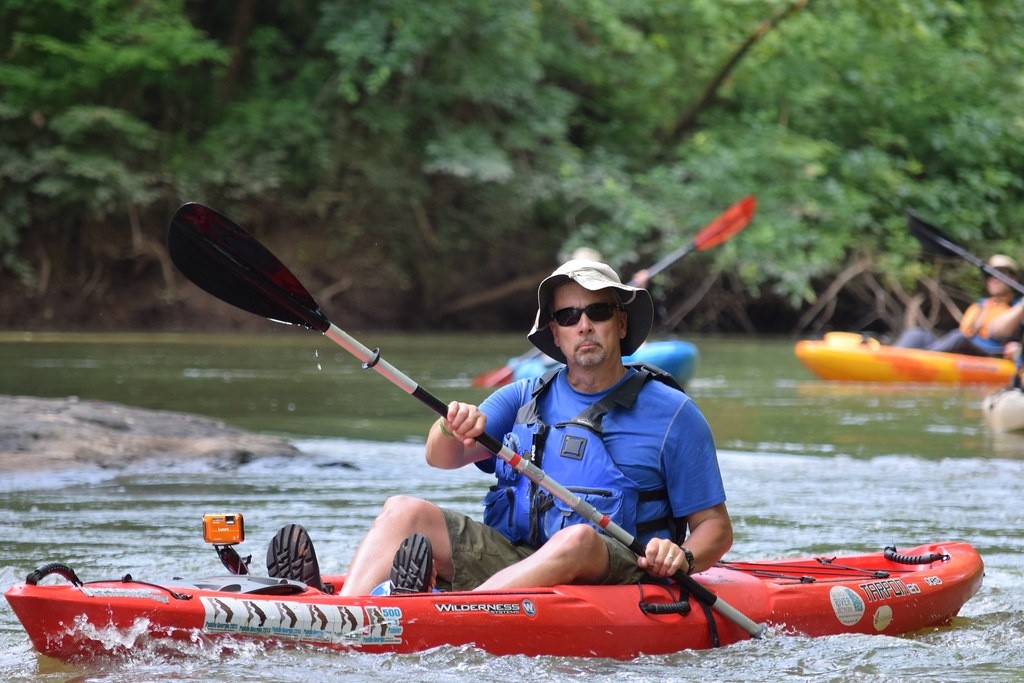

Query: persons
left=267, top=260, right=733, bottom=597
left=891, top=254, right=1024, bottom=358
left=569, top=247, right=650, bottom=290
left=988, top=295, right=1024, bottom=393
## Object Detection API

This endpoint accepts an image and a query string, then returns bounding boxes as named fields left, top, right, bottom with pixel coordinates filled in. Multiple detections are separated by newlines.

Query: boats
left=794, top=332, right=1019, bottom=385
left=5, top=542, right=985, bottom=663
left=509, top=340, right=699, bottom=391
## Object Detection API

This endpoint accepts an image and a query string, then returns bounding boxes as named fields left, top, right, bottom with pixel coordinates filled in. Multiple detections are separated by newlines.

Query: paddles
left=906, top=212, right=1024, bottom=296
left=472, top=194, right=756, bottom=390
left=168, top=201, right=769, bottom=639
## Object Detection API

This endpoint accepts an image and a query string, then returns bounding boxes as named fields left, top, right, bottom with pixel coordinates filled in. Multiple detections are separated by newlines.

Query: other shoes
left=267, top=523, right=322, bottom=591
left=390, top=534, right=438, bottom=594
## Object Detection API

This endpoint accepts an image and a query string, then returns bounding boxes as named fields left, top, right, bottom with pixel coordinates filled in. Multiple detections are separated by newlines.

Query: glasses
left=551, top=301, right=623, bottom=327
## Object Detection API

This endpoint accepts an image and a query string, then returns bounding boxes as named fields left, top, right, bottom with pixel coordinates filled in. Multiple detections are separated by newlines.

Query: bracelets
left=440, top=418, right=455, bottom=440
left=680, top=546, right=695, bottom=574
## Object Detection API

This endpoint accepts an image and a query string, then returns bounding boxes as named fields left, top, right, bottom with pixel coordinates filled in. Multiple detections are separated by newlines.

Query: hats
left=988, top=254, right=1019, bottom=275
left=527, top=259, right=655, bottom=365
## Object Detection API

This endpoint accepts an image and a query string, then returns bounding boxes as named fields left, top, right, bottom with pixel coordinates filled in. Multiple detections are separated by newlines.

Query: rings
left=667, top=553, right=674, bottom=559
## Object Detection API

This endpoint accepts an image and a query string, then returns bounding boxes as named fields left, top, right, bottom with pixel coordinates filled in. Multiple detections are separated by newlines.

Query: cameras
left=203, top=512, right=245, bottom=543
